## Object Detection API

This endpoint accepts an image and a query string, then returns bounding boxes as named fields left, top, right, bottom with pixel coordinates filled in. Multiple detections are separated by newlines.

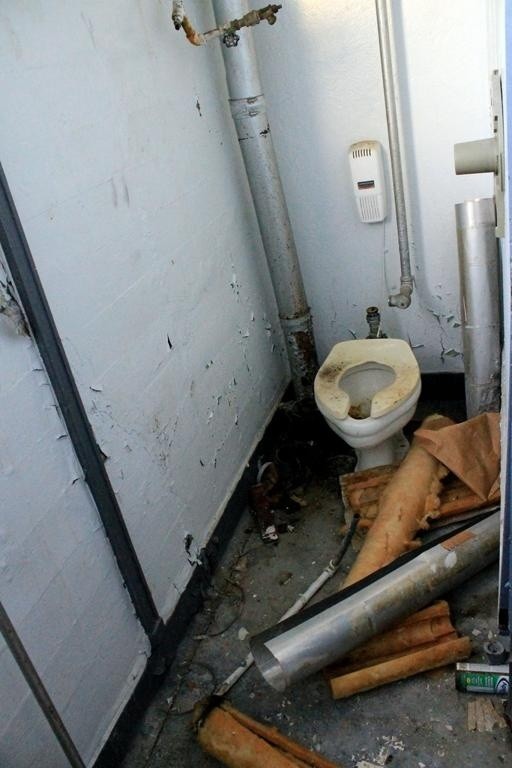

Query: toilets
left=314, top=306, right=422, bottom=473
